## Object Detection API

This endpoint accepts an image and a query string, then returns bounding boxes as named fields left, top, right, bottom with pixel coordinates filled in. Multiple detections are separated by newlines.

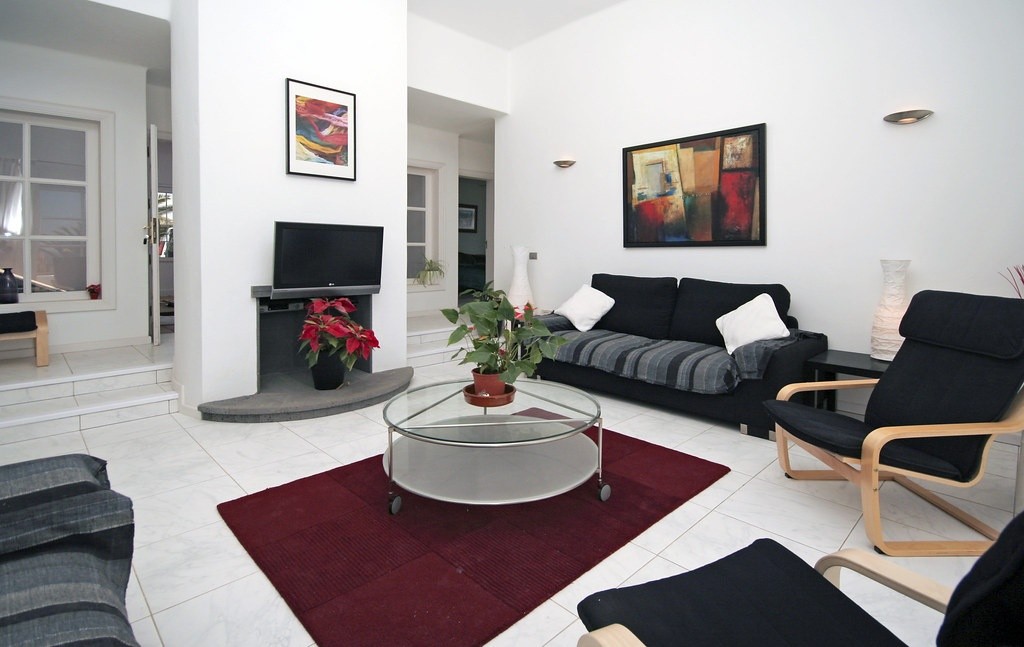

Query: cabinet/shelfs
left=149, top=230, right=175, bottom=329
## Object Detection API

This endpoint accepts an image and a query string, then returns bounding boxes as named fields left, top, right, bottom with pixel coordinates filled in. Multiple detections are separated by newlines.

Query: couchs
left=509, top=272, right=829, bottom=438
left=0, top=454, right=141, bottom=647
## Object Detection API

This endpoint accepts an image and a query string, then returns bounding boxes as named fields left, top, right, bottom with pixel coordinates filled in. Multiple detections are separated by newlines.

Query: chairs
left=576, top=289, right=1024, bottom=647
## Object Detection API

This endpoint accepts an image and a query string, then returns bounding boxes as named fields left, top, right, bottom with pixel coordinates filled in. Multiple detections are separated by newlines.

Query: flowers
left=86, top=284, right=102, bottom=293
left=297, top=298, right=380, bottom=372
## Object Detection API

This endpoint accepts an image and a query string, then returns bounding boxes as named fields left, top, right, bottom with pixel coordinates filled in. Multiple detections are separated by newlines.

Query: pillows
left=717, top=293, right=790, bottom=354
left=554, top=284, right=615, bottom=332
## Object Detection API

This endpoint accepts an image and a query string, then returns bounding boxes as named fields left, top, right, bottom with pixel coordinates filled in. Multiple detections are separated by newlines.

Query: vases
left=0, top=267, right=18, bottom=304
left=311, top=353, right=347, bottom=389
left=90, top=293, right=98, bottom=299
left=508, top=245, right=534, bottom=314
left=870, top=258, right=913, bottom=360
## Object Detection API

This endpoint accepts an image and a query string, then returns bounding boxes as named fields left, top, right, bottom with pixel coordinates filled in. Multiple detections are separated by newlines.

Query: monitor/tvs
left=270, top=221, right=384, bottom=299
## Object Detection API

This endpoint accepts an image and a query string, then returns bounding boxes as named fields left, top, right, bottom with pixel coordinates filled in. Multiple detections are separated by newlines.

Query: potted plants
left=413, top=258, right=571, bottom=407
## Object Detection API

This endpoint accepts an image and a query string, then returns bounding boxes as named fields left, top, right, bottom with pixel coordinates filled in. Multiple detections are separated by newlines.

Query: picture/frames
left=623, top=123, right=769, bottom=248
left=458, top=204, right=478, bottom=232
left=286, top=78, right=356, bottom=181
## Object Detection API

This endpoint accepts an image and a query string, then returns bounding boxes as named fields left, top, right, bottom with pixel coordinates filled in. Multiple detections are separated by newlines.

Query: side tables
left=805, top=349, right=893, bottom=424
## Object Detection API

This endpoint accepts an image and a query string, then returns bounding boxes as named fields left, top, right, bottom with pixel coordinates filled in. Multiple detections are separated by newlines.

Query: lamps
left=554, top=161, right=576, bottom=167
left=883, top=110, right=934, bottom=124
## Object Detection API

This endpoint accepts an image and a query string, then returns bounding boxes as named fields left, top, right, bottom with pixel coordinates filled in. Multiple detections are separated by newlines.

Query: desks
left=382, top=378, right=611, bottom=514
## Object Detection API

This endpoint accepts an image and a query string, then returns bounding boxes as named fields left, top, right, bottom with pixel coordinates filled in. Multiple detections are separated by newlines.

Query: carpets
left=217, top=407, right=731, bottom=647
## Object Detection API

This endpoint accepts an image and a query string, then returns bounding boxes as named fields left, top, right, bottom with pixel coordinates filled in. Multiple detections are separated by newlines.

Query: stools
left=0, top=310, right=49, bottom=367
